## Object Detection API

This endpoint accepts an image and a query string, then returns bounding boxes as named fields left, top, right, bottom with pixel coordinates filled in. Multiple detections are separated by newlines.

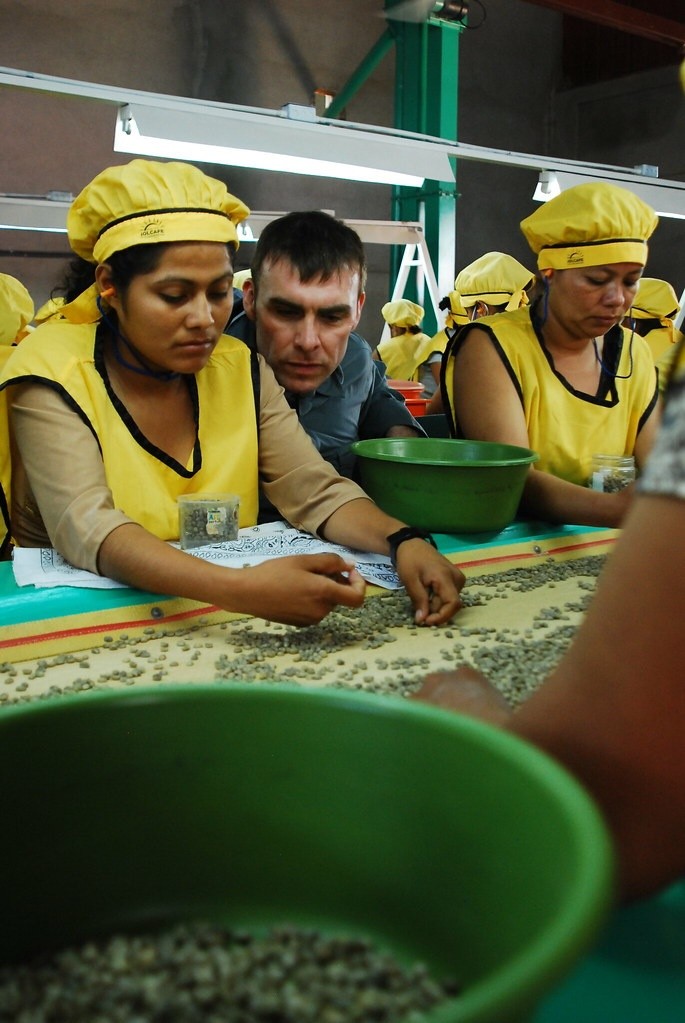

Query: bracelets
left=386, top=526, right=437, bottom=569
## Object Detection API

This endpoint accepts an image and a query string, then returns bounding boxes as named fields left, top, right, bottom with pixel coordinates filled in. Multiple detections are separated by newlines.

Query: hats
left=449, top=292, right=473, bottom=329
left=35, top=297, right=65, bottom=322
left=381, top=299, right=425, bottom=328
left=233, top=270, right=252, bottom=289
left=0, top=273, right=34, bottom=346
left=454, top=251, right=534, bottom=311
left=64, top=160, right=250, bottom=265
left=517, top=183, right=656, bottom=269
left=625, top=277, right=680, bottom=319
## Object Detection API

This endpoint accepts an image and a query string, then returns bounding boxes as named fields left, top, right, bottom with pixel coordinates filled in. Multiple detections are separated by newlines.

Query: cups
left=180, top=493, right=240, bottom=553
left=589, top=454, right=636, bottom=494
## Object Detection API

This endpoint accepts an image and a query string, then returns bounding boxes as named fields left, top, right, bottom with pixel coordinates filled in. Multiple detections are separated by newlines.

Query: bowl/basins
left=387, top=377, right=432, bottom=419
left=0, top=680, right=617, bottom=1023
left=351, top=438, right=542, bottom=532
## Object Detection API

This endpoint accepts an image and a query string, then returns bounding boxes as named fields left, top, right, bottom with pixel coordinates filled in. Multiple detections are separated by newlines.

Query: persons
left=371, top=298, right=431, bottom=382
left=438, top=183, right=661, bottom=530
left=412, top=378, right=685, bottom=908
left=0, top=159, right=469, bottom=629
left=430, top=287, right=470, bottom=385
left=622, top=276, right=685, bottom=395
left=455, top=251, right=535, bottom=322
left=0, top=271, right=36, bottom=559
left=222, top=211, right=429, bottom=473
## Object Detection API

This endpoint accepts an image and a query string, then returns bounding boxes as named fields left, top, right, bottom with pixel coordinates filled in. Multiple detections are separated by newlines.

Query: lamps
left=113, top=103, right=457, bottom=188
left=532, top=171, right=684, bottom=219
